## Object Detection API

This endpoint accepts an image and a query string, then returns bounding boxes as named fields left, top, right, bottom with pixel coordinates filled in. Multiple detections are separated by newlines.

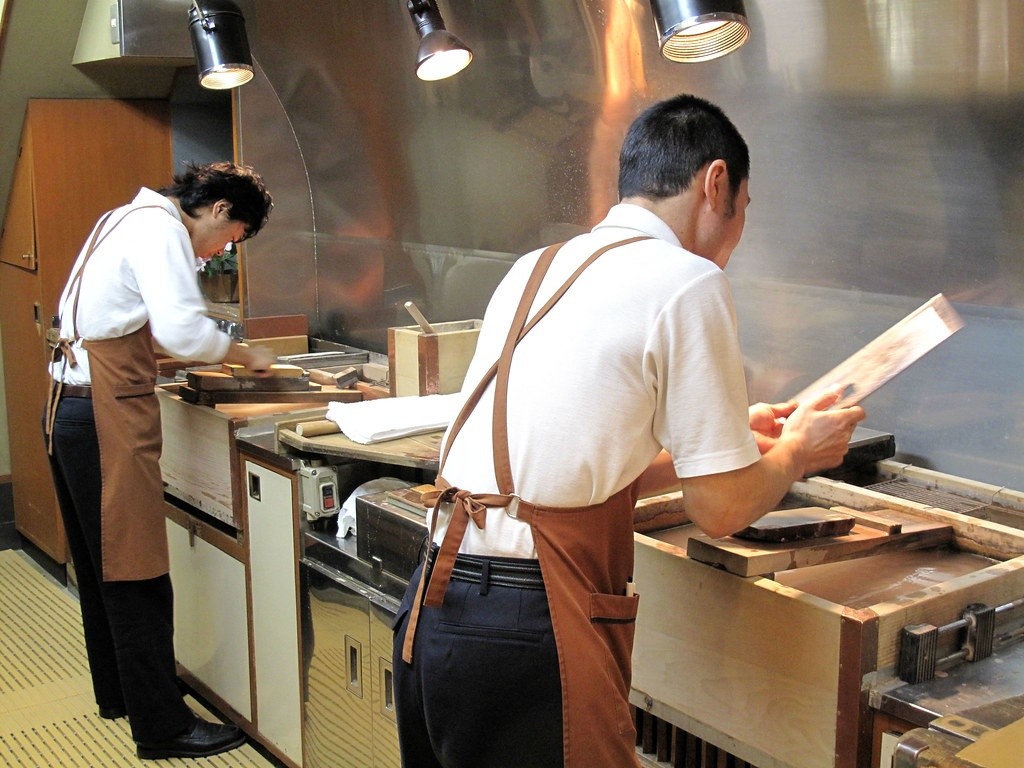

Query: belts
left=53, top=382, right=92, bottom=398
left=421, top=539, right=545, bottom=589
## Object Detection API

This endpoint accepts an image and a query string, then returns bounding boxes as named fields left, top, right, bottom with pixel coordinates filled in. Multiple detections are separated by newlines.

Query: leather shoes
left=98, top=676, right=189, bottom=719
left=136, top=715, right=246, bottom=759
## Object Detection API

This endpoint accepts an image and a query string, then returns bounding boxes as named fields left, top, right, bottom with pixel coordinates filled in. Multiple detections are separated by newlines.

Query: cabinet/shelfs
left=145, top=384, right=305, bottom=767
left=1, top=94, right=173, bottom=582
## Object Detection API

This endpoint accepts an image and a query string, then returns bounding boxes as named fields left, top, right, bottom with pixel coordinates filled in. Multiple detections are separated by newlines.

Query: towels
left=325, top=391, right=461, bottom=445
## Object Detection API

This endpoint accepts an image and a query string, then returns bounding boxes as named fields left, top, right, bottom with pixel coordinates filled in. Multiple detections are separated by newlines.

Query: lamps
left=188, top=0, right=255, bottom=90
left=650, top=0, right=750, bottom=64
left=407, top=0, right=472, bottom=81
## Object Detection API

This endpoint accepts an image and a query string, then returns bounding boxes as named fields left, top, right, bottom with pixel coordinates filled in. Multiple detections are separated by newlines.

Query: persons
left=42, top=160, right=277, bottom=759
left=392, top=93, right=866, bottom=768
